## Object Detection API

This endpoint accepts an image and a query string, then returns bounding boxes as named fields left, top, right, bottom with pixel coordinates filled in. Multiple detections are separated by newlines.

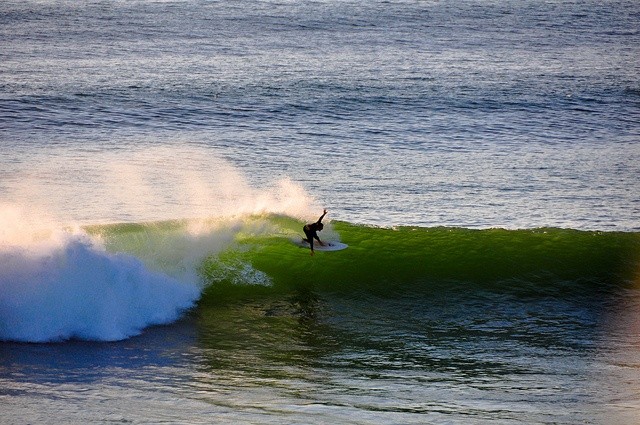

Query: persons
left=303, top=210, right=328, bottom=256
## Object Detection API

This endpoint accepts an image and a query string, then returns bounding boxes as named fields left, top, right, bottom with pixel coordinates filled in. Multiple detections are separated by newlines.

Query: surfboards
left=304, top=239, right=349, bottom=252
